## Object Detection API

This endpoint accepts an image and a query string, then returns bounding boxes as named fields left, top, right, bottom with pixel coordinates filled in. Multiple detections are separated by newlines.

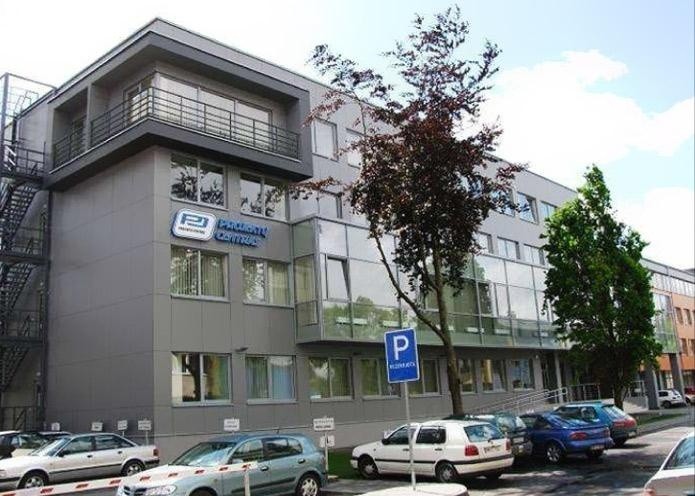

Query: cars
left=658, top=386, right=695, bottom=409
left=644, top=431, right=695, bottom=496
left=349, top=412, right=533, bottom=484
left=116, top=433, right=328, bottom=496
left=0, top=429, right=160, bottom=492
left=519, top=400, right=638, bottom=463
